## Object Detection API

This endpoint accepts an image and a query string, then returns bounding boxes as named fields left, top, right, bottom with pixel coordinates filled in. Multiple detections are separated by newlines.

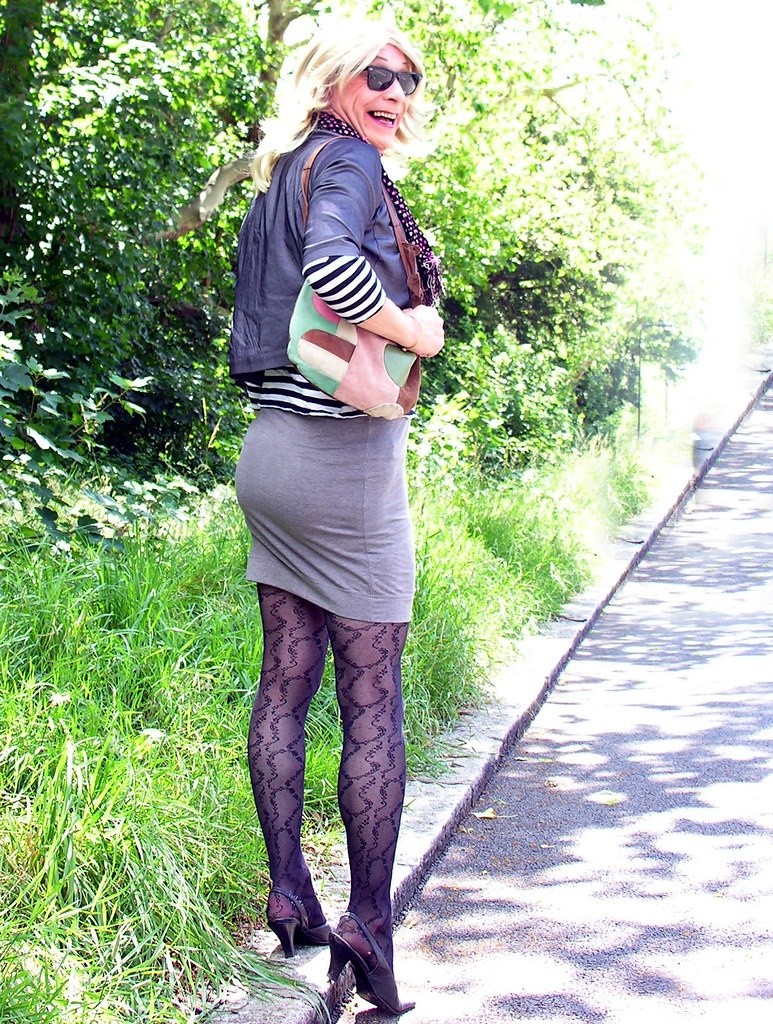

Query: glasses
left=354, top=65, right=423, bottom=96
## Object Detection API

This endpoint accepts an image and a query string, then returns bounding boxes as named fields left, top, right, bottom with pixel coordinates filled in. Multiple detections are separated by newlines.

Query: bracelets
left=400, top=310, right=422, bottom=351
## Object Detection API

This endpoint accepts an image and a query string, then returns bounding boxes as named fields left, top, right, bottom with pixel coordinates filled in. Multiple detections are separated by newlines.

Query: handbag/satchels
left=286, top=135, right=421, bottom=421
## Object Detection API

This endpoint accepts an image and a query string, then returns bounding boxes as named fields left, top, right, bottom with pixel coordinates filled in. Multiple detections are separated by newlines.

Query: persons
left=233, top=15, right=445, bottom=1017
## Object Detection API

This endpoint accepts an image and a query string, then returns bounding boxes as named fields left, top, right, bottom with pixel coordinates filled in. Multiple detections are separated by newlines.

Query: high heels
left=327, top=913, right=416, bottom=1014
left=267, top=887, right=333, bottom=958
